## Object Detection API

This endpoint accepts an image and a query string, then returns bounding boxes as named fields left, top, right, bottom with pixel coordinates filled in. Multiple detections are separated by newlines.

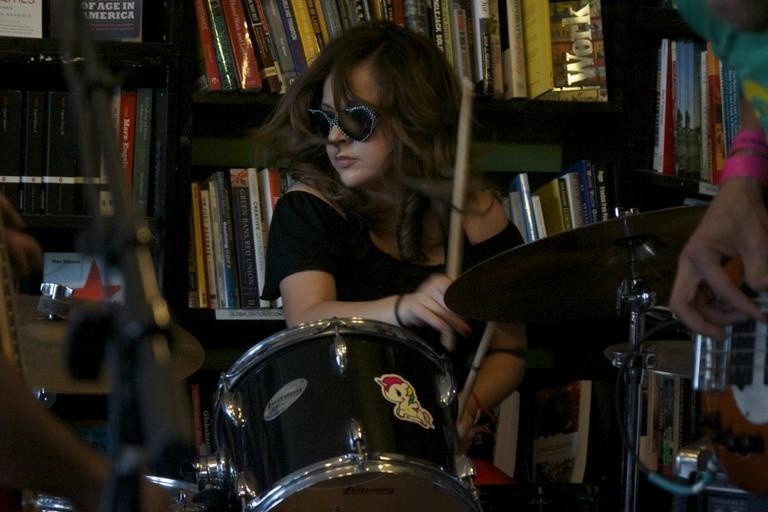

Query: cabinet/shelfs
left=0, top=0, right=768, bottom=511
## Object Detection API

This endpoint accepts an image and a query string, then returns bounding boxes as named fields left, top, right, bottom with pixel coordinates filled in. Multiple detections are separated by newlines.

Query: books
left=649, top=35, right=741, bottom=196
left=198, top=383, right=215, bottom=453
left=463, top=388, right=520, bottom=485
left=189, top=378, right=202, bottom=452
left=499, top=156, right=615, bottom=249
left=182, top=0, right=608, bottom=102
left=627, top=368, right=767, bottom=512
left=527, top=378, right=593, bottom=484
left=187, top=164, right=299, bottom=308
left=0, top=0, right=155, bottom=218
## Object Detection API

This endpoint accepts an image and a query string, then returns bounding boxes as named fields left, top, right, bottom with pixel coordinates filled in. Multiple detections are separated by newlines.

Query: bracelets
left=717, top=152, right=767, bottom=184
left=394, top=291, right=409, bottom=331
left=470, top=391, right=485, bottom=417
left=729, top=129, right=766, bottom=155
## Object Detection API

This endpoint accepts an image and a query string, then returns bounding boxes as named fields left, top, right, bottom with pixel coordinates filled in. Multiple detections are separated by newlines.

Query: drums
left=22, top=475, right=210, bottom=512
left=211, top=316, right=482, bottom=511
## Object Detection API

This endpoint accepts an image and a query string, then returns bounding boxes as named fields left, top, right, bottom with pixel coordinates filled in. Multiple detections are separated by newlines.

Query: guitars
left=692, top=254, right=768, bottom=497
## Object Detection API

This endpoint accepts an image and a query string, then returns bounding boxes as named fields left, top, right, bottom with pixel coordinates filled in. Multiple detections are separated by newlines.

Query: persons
left=0, top=339, right=175, bottom=511
left=666, top=0, right=766, bottom=343
left=255, top=16, right=532, bottom=510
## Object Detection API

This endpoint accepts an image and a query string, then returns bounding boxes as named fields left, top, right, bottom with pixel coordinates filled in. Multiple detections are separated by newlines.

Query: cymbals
left=0, top=294, right=206, bottom=394
left=603, top=340, right=692, bottom=380
left=444, top=204, right=745, bottom=333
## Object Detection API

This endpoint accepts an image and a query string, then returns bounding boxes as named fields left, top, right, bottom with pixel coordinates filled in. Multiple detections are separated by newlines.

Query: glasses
left=307, top=106, right=376, bottom=143
